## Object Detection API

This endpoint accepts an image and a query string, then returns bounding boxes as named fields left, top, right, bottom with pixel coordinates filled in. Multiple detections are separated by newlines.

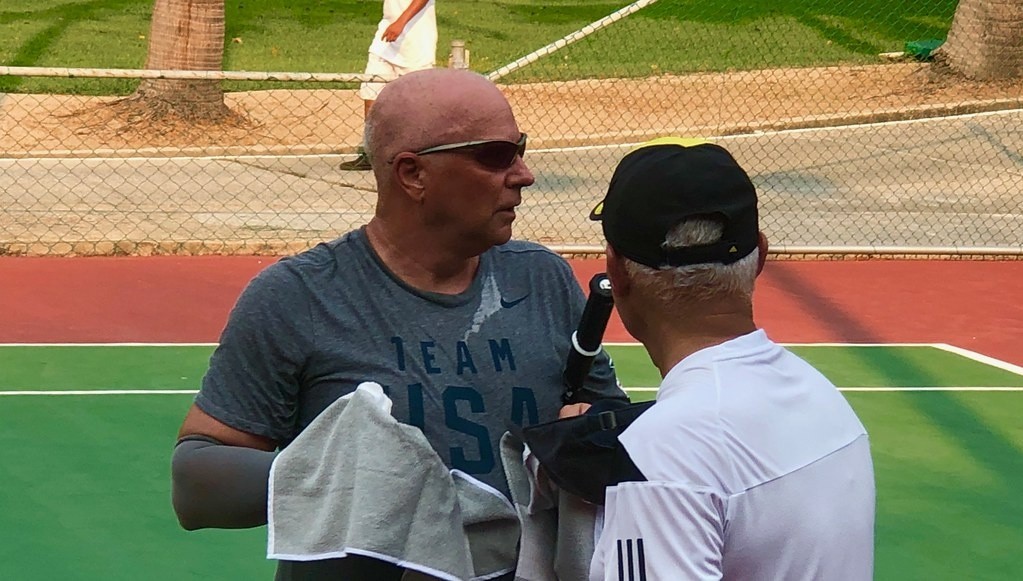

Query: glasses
left=387, top=132, right=528, bottom=171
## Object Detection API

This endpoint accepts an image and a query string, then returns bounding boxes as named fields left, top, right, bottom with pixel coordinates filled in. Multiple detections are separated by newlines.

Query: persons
left=585, top=136, right=877, bottom=581
left=171, top=67, right=633, bottom=581
left=339, top=0, right=439, bottom=170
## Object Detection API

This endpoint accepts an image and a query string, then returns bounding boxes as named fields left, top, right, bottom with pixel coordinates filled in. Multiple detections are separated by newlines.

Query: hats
left=589, top=144, right=760, bottom=271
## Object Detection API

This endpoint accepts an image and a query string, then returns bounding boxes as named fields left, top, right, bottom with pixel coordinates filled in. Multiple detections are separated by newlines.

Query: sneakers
left=340, top=153, right=372, bottom=171
